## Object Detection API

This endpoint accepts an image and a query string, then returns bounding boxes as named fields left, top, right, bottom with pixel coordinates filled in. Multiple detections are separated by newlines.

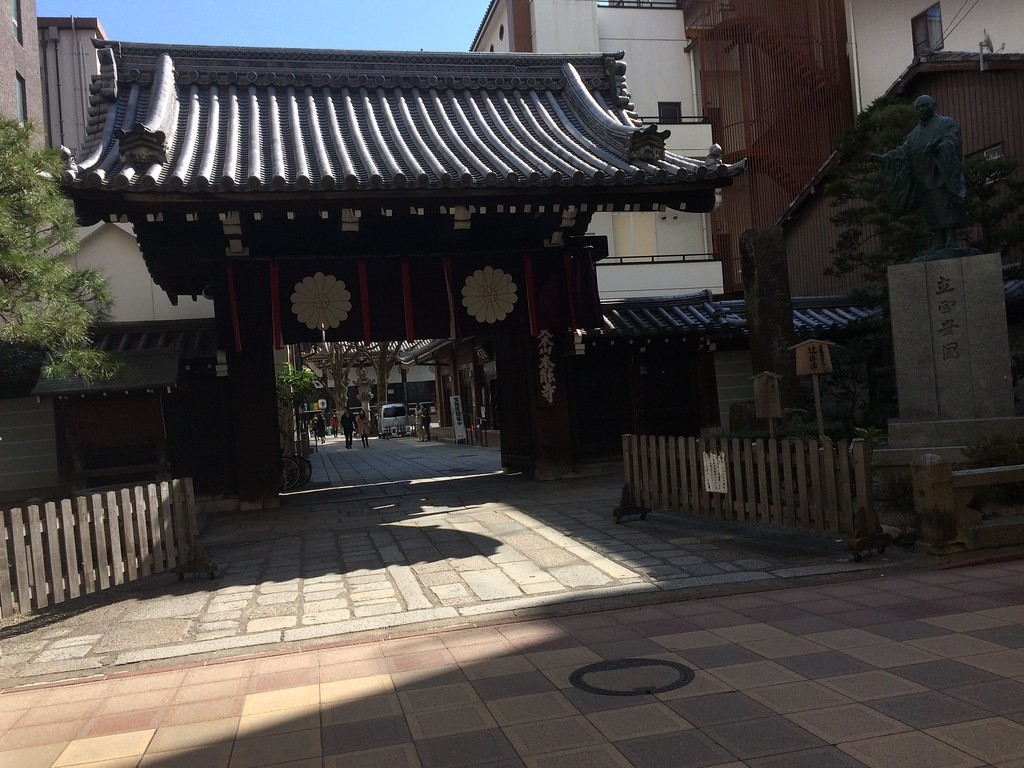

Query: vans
left=378, top=404, right=408, bottom=439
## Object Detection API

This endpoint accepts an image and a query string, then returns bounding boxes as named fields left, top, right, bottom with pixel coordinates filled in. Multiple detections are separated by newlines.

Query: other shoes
left=364, top=444, right=365, bottom=448
left=417, top=439, right=424, bottom=442
left=346, top=445, right=349, bottom=449
left=426, top=439, right=431, bottom=442
left=367, top=444, right=369, bottom=447
left=350, top=444, right=352, bottom=449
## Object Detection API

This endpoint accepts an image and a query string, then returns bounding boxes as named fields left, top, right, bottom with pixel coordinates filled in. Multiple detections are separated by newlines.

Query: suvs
left=408, top=403, right=418, bottom=413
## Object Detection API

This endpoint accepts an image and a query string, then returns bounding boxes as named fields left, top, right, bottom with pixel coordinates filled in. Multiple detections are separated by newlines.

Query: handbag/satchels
left=422, top=417, right=429, bottom=426
left=367, top=423, right=371, bottom=427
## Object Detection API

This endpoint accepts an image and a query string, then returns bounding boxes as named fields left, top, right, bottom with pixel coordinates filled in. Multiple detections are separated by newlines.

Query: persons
left=414, top=404, right=431, bottom=442
left=331, top=413, right=339, bottom=438
left=339, top=407, right=358, bottom=449
left=356, top=410, right=370, bottom=448
left=315, top=414, right=326, bottom=443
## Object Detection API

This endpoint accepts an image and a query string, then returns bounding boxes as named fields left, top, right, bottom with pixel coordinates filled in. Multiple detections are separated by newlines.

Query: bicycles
left=290, top=455, right=312, bottom=487
left=278, top=457, right=299, bottom=490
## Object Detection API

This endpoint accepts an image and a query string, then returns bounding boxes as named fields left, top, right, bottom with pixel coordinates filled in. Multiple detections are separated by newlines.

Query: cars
left=418, top=402, right=437, bottom=414
